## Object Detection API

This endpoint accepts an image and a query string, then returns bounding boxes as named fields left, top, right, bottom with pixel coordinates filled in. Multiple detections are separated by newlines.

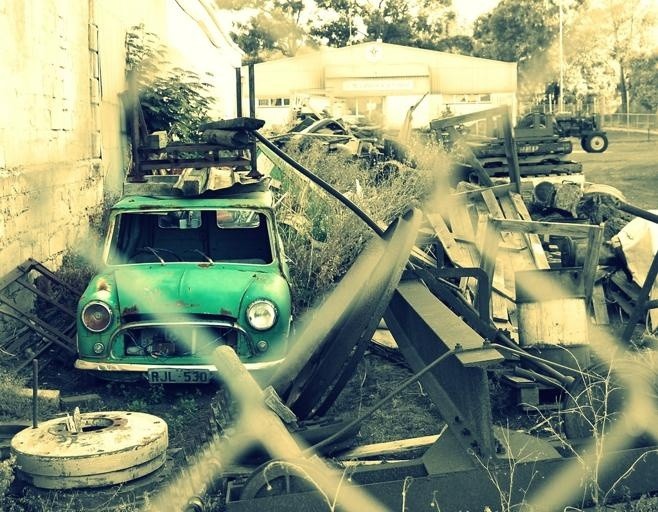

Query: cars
left=74, top=183, right=293, bottom=385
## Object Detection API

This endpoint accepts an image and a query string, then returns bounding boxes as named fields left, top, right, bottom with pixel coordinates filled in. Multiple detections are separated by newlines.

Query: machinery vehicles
left=516, top=113, right=608, bottom=154
